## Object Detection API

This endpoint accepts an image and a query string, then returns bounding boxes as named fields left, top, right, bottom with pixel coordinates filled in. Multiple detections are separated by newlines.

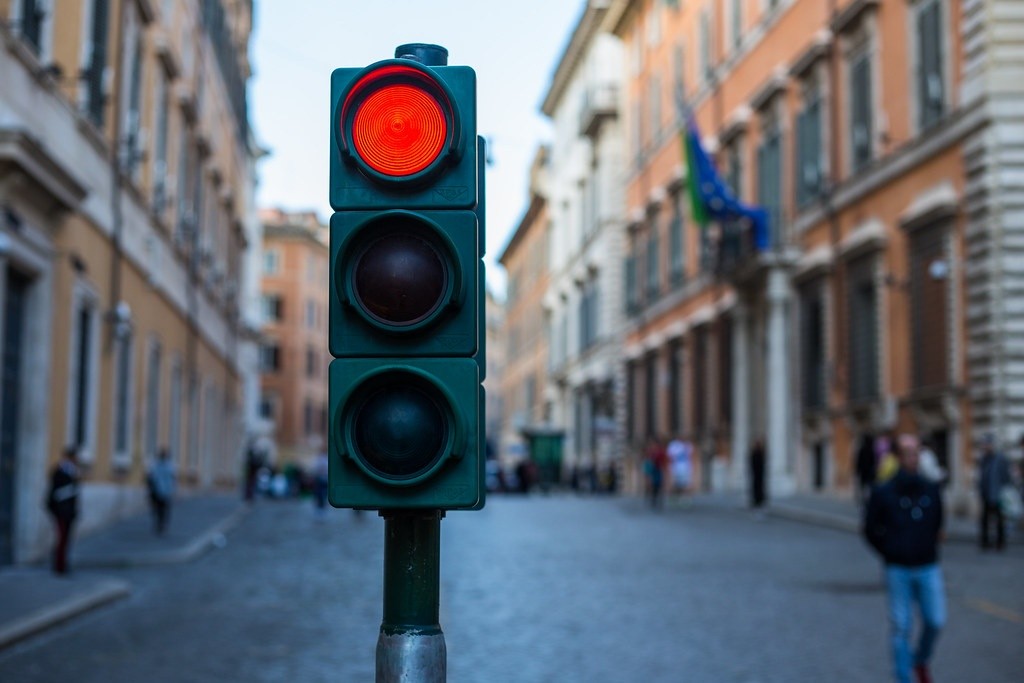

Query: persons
left=667, top=439, right=694, bottom=495
left=854, top=437, right=946, bottom=509
left=861, top=434, right=947, bottom=683
left=150, top=448, right=175, bottom=536
left=641, top=439, right=666, bottom=503
left=45, top=445, right=81, bottom=575
left=750, top=439, right=765, bottom=507
left=977, top=434, right=1008, bottom=550
left=246, top=440, right=258, bottom=500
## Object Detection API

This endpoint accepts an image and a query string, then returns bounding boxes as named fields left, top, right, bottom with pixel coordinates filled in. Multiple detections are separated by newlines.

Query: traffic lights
left=328, top=57, right=488, bottom=510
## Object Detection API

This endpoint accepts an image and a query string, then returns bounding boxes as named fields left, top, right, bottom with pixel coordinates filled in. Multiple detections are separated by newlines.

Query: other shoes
left=913, top=664, right=933, bottom=683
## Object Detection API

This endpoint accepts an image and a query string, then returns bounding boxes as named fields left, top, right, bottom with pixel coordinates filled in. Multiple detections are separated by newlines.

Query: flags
left=680, top=105, right=770, bottom=256
